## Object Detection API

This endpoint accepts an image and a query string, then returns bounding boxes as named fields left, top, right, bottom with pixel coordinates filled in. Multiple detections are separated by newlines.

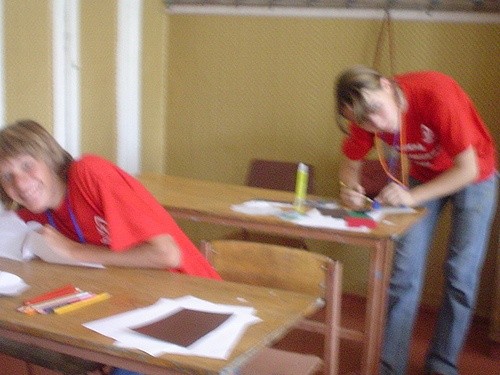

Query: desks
left=0, top=258, right=324, bottom=374
left=121, top=173, right=430, bottom=375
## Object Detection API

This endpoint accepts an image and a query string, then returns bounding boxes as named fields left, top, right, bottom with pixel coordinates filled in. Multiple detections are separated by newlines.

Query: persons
left=333, top=63, right=500, bottom=375
left=0, top=118, right=224, bottom=281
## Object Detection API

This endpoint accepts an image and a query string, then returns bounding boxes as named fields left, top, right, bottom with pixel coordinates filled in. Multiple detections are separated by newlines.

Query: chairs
left=219, top=160, right=314, bottom=249
left=200, top=240, right=341, bottom=375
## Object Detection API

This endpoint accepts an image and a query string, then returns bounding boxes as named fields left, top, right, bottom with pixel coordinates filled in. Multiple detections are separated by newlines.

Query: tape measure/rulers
left=53, top=292, right=112, bottom=316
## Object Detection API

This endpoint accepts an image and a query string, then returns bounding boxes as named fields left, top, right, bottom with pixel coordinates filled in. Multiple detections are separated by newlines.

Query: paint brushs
left=337, top=180, right=375, bottom=205
